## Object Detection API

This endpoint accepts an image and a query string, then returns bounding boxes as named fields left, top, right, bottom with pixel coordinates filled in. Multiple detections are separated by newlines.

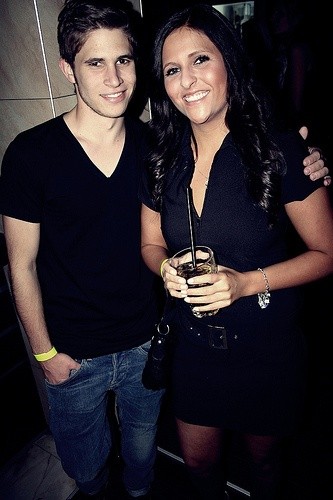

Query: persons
left=141, top=6, right=333, bottom=500
left=0, top=0, right=333, bottom=500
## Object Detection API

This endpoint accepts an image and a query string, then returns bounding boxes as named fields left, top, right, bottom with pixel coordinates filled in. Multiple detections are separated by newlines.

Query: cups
left=171, top=246, right=219, bottom=318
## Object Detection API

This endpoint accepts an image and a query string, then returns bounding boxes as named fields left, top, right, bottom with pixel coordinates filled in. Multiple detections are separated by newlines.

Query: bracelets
left=159, top=257, right=169, bottom=282
left=257, top=266, right=273, bottom=309
left=33, top=347, right=59, bottom=365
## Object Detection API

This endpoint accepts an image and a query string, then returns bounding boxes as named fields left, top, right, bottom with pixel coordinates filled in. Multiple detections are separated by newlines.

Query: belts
left=176, top=303, right=249, bottom=349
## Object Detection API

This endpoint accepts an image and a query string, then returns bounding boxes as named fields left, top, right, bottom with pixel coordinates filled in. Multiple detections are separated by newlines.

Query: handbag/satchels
left=142, top=334, right=175, bottom=391
